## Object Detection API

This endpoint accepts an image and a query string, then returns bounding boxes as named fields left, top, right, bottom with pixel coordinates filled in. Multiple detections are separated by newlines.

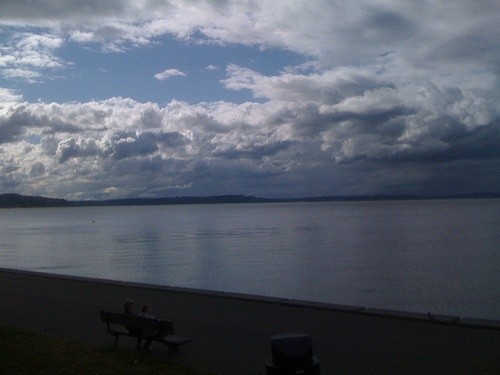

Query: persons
left=135, top=304, right=157, bottom=351
left=124, top=300, right=137, bottom=334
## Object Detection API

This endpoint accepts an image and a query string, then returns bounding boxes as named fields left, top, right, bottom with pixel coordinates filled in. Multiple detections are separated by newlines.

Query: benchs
left=98, top=308, right=193, bottom=361
